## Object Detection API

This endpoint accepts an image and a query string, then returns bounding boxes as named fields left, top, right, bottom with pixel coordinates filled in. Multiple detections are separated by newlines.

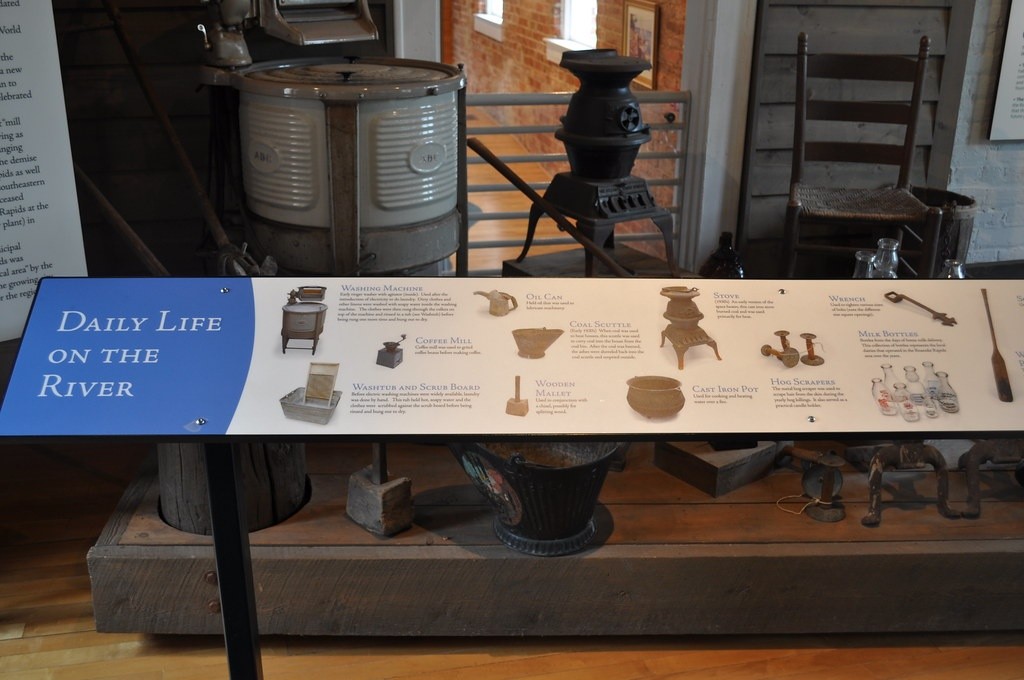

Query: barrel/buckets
left=451, top=441, right=618, bottom=555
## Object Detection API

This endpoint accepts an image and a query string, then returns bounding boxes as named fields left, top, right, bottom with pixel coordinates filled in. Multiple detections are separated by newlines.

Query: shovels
left=981, top=288, right=1014, bottom=402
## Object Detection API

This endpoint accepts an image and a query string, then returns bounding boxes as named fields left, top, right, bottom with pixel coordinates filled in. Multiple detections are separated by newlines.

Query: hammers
left=346, top=443, right=414, bottom=535
left=506, top=376, right=530, bottom=416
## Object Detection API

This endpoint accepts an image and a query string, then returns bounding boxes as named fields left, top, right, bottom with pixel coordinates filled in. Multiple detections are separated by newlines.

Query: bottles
left=703, top=232, right=747, bottom=279
left=853, top=250, right=876, bottom=279
left=938, top=259, right=966, bottom=279
left=872, top=362, right=959, bottom=422
left=877, top=238, right=900, bottom=279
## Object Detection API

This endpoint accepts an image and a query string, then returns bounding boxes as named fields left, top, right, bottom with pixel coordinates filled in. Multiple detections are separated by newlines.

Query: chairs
left=784, top=30, right=945, bottom=279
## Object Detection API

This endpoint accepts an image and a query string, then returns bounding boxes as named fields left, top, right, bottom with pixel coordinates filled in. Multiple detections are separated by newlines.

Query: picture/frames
left=620, top=0, right=658, bottom=92
